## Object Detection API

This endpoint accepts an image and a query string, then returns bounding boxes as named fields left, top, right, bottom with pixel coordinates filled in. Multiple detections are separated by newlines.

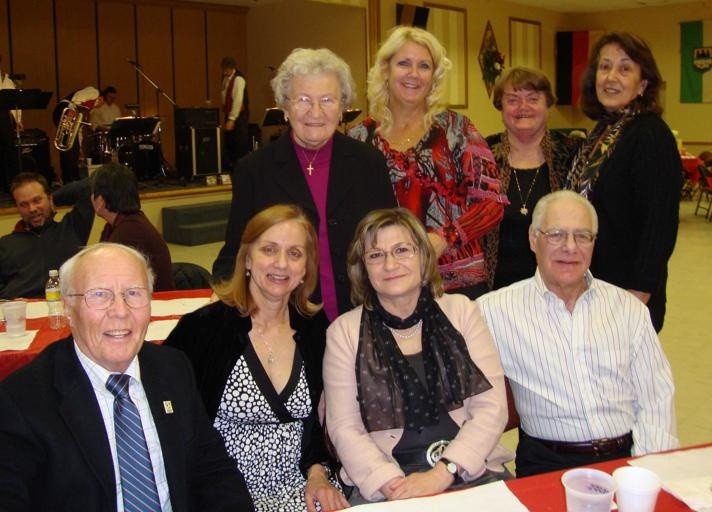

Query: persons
left=340, top=25, right=510, bottom=301
left=87, top=160, right=172, bottom=273
left=52, top=86, right=108, bottom=182
left=482, top=64, right=590, bottom=292
left=0, top=67, right=25, bottom=135
left=160, top=202, right=359, bottom=512
left=1, top=243, right=259, bottom=511
left=319, top=207, right=512, bottom=500
left=219, top=56, right=251, bottom=144
left=472, top=189, right=682, bottom=478
left=1, top=170, right=96, bottom=297
left=88, top=85, right=123, bottom=133
left=208, top=43, right=400, bottom=321
left=560, top=24, right=683, bottom=337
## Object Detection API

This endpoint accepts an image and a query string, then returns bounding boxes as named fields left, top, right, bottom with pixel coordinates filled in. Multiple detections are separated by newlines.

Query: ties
left=105, top=375, right=161, bottom=512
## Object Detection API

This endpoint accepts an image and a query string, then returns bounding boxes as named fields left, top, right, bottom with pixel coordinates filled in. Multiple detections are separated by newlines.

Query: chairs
left=680, top=150, right=712, bottom=221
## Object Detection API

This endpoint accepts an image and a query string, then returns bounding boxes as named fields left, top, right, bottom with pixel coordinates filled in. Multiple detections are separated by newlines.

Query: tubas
left=55, top=104, right=91, bottom=151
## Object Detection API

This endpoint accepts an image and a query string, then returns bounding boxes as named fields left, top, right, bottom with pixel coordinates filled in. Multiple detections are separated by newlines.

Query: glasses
left=287, top=95, right=335, bottom=106
left=68, top=288, right=148, bottom=310
left=538, top=229, right=594, bottom=243
left=364, top=246, right=414, bottom=264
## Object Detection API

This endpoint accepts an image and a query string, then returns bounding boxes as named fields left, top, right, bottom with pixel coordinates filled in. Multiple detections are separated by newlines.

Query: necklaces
left=508, top=154, right=544, bottom=217
left=397, top=122, right=418, bottom=145
left=382, top=321, right=426, bottom=340
left=254, top=325, right=292, bottom=364
left=293, top=136, right=329, bottom=176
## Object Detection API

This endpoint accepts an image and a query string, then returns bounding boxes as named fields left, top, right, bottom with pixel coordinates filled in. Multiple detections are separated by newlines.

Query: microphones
left=125, top=56, right=143, bottom=68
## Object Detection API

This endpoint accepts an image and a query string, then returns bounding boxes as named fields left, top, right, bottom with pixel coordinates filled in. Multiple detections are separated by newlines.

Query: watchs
left=439, top=456, right=461, bottom=485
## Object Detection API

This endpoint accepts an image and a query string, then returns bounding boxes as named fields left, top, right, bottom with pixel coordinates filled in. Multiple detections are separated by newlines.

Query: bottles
left=45, top=269, right=67, bottom=330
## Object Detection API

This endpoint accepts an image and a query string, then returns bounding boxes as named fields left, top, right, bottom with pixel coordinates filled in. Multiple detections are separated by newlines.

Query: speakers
left=174, top=107, right=221, bottom=181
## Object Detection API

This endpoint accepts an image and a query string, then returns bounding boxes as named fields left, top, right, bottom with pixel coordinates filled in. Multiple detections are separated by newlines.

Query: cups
left=613, top=465, right=664, bottom=511
left=560, top=467, right=618, bottom=512
left=2, top=300, right=27, bottom=337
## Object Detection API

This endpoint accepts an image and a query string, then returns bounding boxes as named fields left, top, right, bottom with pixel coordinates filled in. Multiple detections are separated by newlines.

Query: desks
left=0, top=286, right=218, bottom=382
left=337, top=443, right=712, bottom=512
